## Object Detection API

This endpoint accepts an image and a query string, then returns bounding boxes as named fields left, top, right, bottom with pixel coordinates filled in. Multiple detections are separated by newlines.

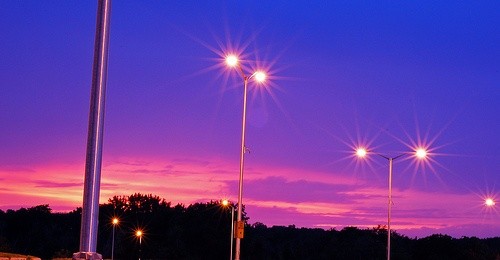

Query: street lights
left=354, top=144, right=432, bottom=260
left=110, top=217, right=118, bottom=259
left=223, top=52, right=268, bottom=260
left=221, top=199, right=235, bottom=260
left=135, top=226, right=144, bottom=260
